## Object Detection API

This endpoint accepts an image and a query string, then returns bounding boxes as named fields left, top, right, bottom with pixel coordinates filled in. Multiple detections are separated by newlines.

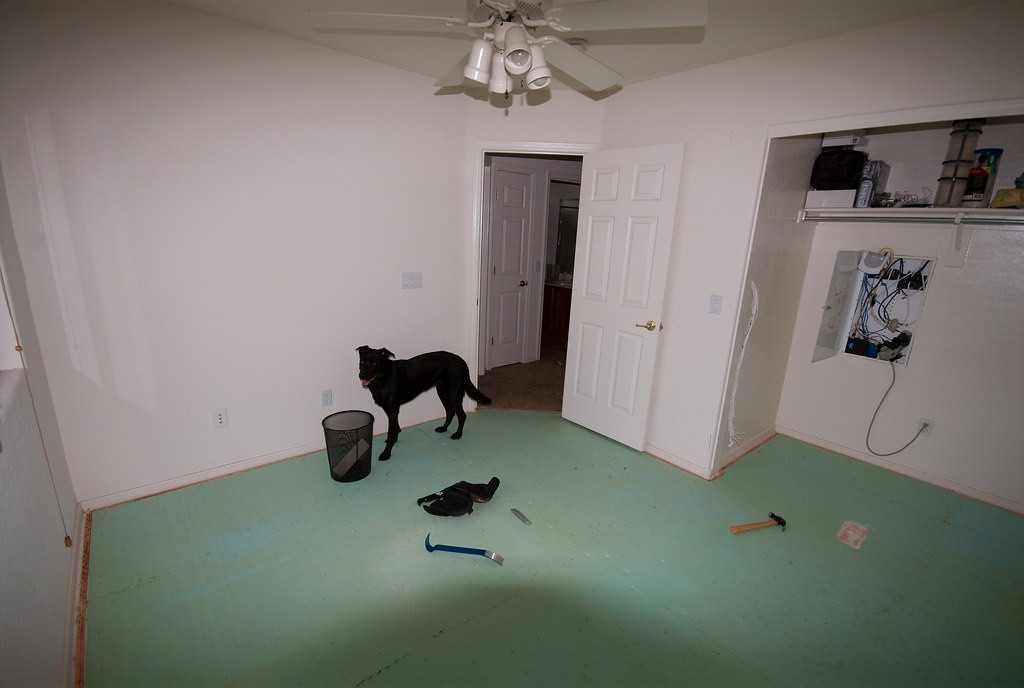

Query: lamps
left=464, top=22, right=553, bottom=101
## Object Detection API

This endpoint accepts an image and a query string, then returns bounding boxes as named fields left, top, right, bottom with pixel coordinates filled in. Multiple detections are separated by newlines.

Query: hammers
left=729, top=511, right=786, bottom=534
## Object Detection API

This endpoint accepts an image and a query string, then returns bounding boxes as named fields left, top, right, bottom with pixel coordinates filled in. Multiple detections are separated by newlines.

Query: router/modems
left=858, top=251, right=888, bottom=275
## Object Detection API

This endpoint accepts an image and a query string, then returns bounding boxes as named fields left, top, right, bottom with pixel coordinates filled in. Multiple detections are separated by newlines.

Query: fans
left=306, top=0, right=710, bottom=92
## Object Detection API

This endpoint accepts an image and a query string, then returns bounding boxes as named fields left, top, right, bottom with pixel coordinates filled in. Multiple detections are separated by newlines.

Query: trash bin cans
left=322, top=410, right=375, bottom=483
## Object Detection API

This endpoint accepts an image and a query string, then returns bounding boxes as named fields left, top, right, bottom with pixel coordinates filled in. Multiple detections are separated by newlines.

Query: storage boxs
left=855, top=159, right=891, bottom=208
left=805, top=190, right=857, bottom=207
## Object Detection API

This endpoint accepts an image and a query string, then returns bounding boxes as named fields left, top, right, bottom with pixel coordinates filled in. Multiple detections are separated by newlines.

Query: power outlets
left=212, top=408, right=228, bottom=428
left=321, top=389, right=333, bottom=407
left=917, top=418, right=934, bottom=437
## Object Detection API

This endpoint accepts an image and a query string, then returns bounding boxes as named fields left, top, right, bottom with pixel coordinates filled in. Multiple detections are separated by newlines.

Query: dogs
left=354, top=345, right=492, bottom=461
